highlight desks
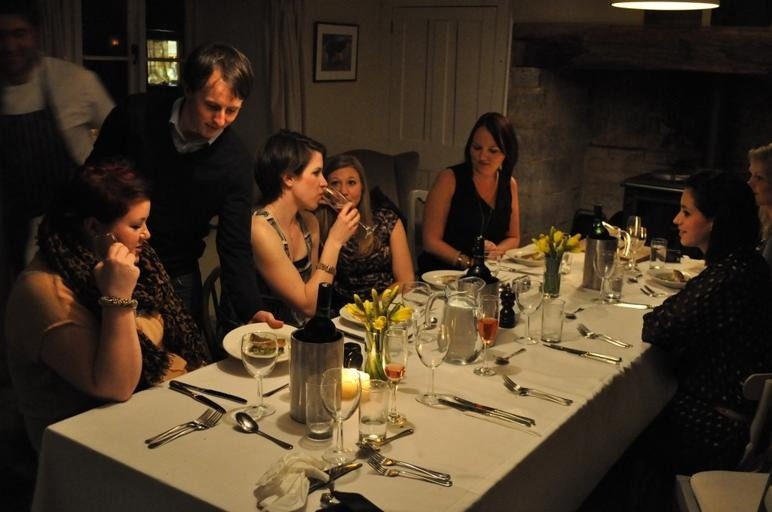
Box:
[623,170,695,247]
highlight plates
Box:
[222,321,301,364]
[421,270,466,290]
[645,267,688,289]
[505,248,544,267]
[339,303,366,327]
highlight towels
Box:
[253,450,330,511]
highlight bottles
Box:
[590,202,610,239]
[302,281,337,342]
[467,231,492,285]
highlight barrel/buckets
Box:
[583,236,618,289]
[288,329,343,424]
[459,276,500,321]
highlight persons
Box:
[619,171,772,512]
[82,43,284,329]
[311,153,416,319]
[746,142,772,268]
[417,112,521,283]
[212,127,361,361]
[2,163,209,453]
[0,0,118,298]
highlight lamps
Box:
[605,0,723,12]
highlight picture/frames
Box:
[312,21,362,82]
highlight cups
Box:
[599,262,625,304]
[559,233,572,274]
[649,237,668,269]
[540,298,566,344]
[358,379,393,447]
[304,372,337,444]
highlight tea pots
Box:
[424,276,487,366]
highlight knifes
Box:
[453,395,536,425]
[542,342,622,365]
[438,397,531,428]
[176,382,248,404]
[613,301,662,310]
[307,463,362,493]
[169,380,227,415]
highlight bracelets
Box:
[98,296,139,310]
[455,251,476,271]
[315,262,338,276]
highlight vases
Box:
[361,330,388,390]
[542,256,562,300]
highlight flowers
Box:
[530,225,583,294]
[344,285,415,381]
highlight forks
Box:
[368,458,453,487]
[501,373,574,404]
[502,381,570,406]
[148,408,223,450]
[143,408,213,445]
[577,322,633,349]
[364,444,450,481]
[640,285,672,299]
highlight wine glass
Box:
[241,331,279,418]
[514,278,543,346]
[320,186,378,238]
[319,368,362,467]
[381,329,409,426]
[415,323,450,406]
[627,215,642,228]
[402,281,433,345]
[591,248,616,305]
[90,233,120,264]
[473,295,500,377]
[623,227,648,275]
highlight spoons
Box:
[496,348,527,365]
[564,307,584,320]
[628,273,644,283]
[354,427,414,450]
[234,412,294,452]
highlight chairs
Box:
[405,186,430,271]
[675,365,772,511]
[196,261,225,345]
